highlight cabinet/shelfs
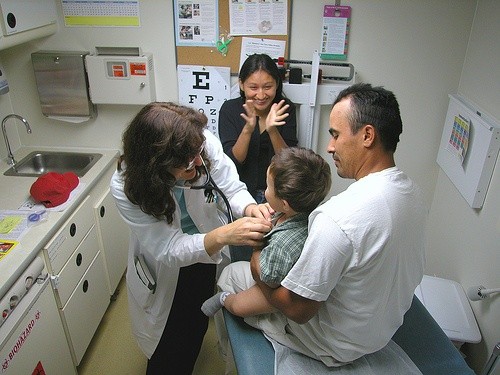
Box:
[41,160,130,369]
[0,0,59,51]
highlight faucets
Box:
[1,113,33,173]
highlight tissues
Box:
[31,49,99,124]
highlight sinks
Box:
[3,150,104,179]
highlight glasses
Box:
[170,138,206,171]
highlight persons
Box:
[109,101,276,375]
[218,52,300,262]
[217,83,425,369]
[201,146,331,318]
